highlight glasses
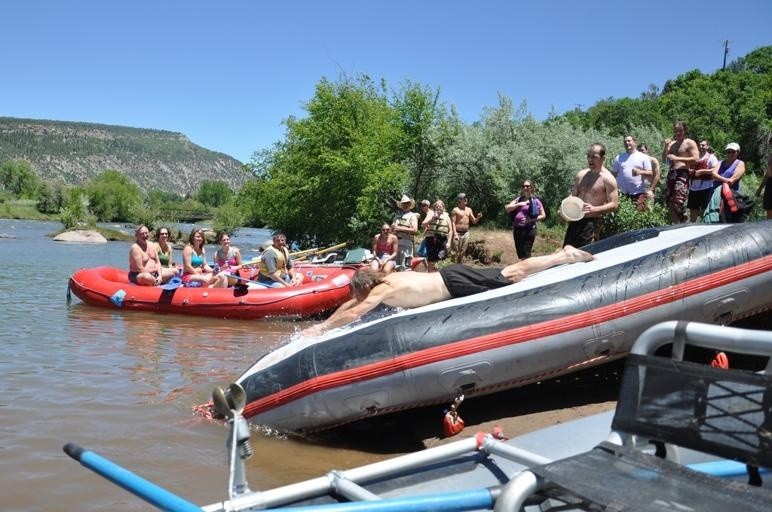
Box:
[522,185,531,189]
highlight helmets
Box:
[559,196,587,222]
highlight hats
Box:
[726,143,739,151]
[419,200,431,207]
[458,192,469,199]
[397,196,416,210]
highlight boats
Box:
[68,264,358,319]
[213,220,770,439]
[167,370,772,509]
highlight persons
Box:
[609,115,771,225]
[370,191,484,274]
[298,242,595,341]
[128,224,305,290]
[563,143,619,248]
[505,179,547,260]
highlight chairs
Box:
[491,317,771,511]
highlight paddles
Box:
[156,281,183,291]
